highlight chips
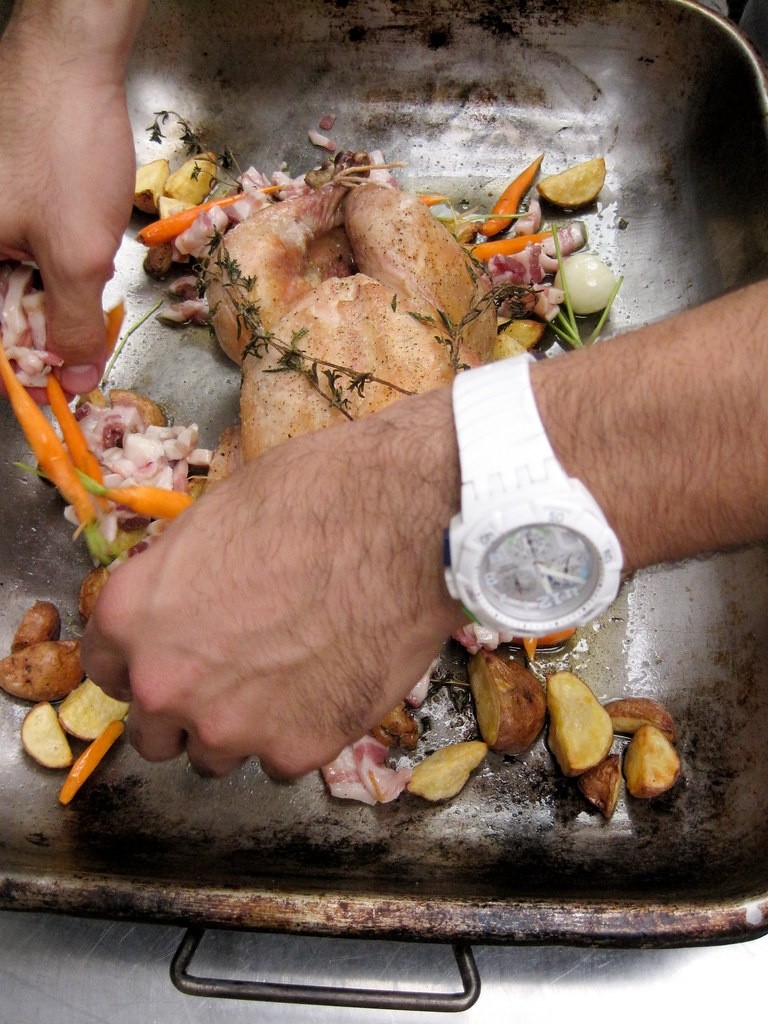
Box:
[132,146,219,215]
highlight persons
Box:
[0,2,768,788]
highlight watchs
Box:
[443,350,628,650]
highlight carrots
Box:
[0,296,207,566]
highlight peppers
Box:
[466,150,557,263]
[137,183,288,243]
[57,721,128,805]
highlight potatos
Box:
[406,647,679,820]
[0,598,133,770]
